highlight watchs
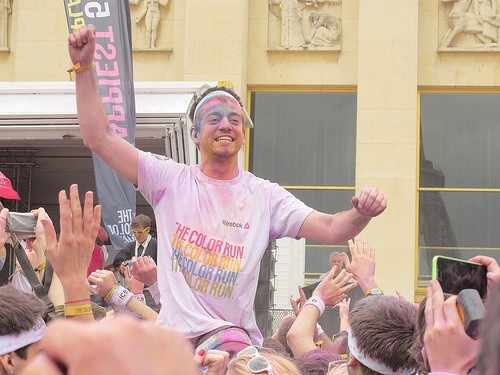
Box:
[363,288,383,297]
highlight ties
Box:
[138,246,144,257]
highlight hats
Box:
[97,226,108,243]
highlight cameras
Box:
[5,212,38,234]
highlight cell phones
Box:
[432,255,488,302]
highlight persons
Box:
[0,173,500,375]
[67,24,387,346]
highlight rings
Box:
[0,216,6,221]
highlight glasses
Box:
[130,227,148,235]
[236,346,274,375]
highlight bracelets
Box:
[67,61,95,81]
[104,285,117,303]
[64,305,92,316]
[65,297,91,304]
[35,260,46,273]
